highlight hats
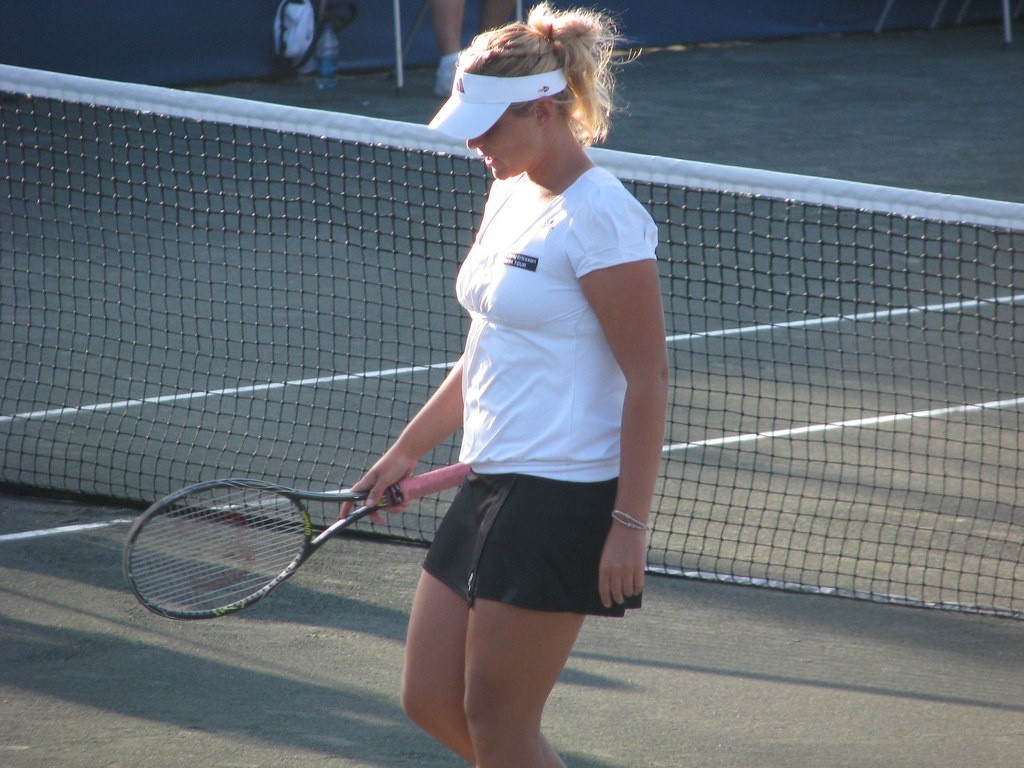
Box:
[426,64,570,142]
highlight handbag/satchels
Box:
[273,0,358,76]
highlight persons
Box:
[341,0,670,768]
[430,0,515,97]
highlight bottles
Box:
[315,21,340,89]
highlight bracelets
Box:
[611,510,651,530]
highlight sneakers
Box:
[433,67,456,97]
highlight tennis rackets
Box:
[125,458,478,623]
